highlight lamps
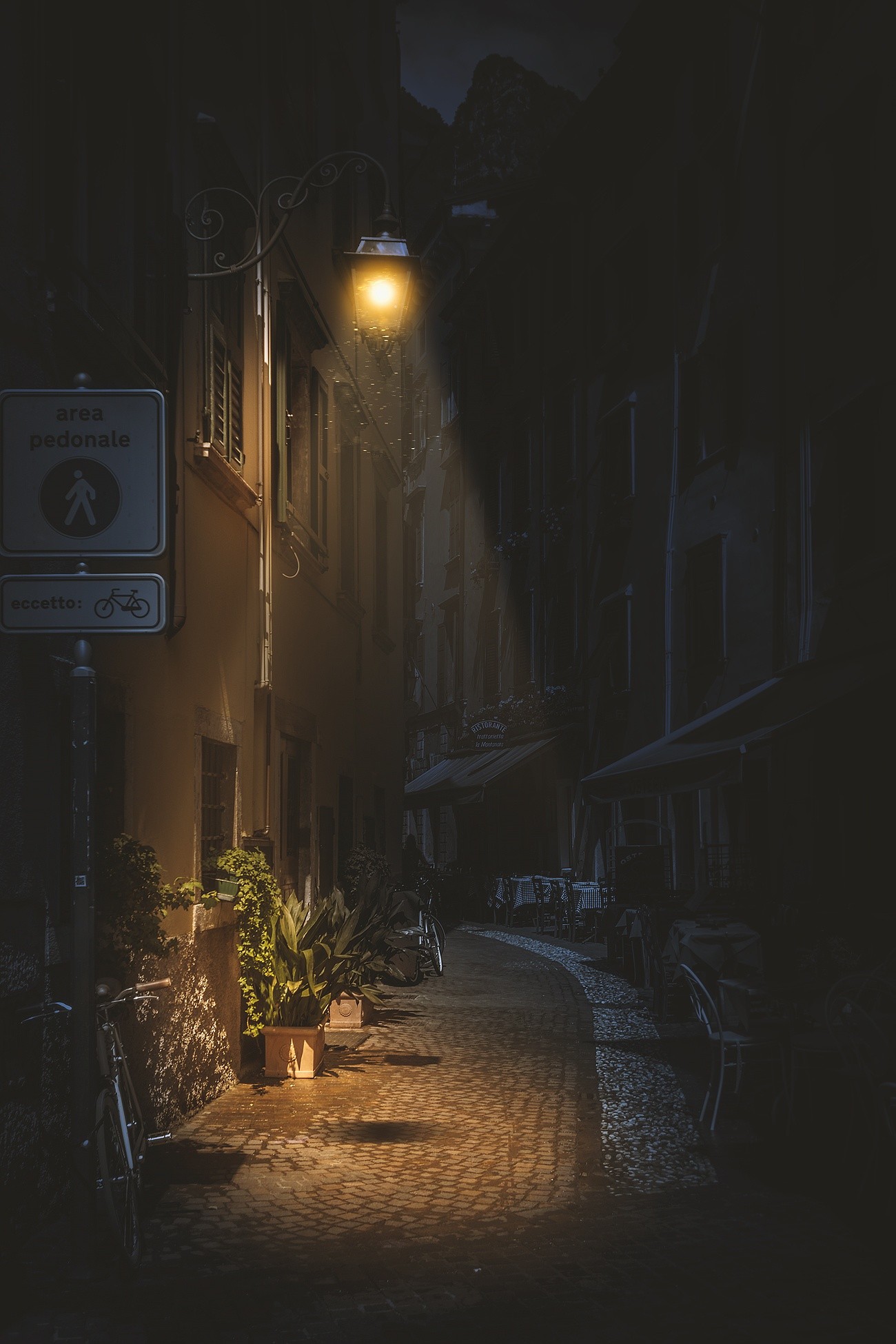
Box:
[153,146,424,367]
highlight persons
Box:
[401,834,429,888]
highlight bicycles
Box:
[15,978,173,1271]
[406,880,446,984]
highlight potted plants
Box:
[258,883,379,1075]
[312,883,409,1028]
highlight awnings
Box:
[405,734,567,804]
[580,676,819,805]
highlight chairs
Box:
[503,876,615,944]
[620,903,896,1133]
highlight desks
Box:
[488,875,616,941]
[715,978,781,1011]
[671,919,762,979]
